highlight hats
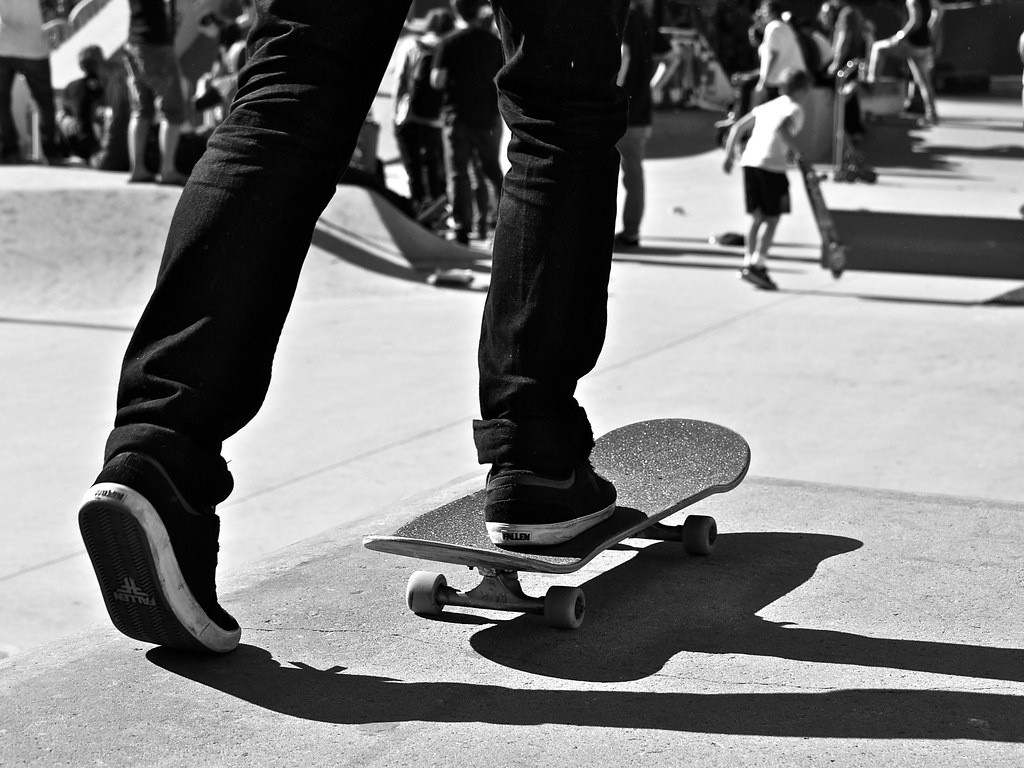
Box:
[407,7,455,36]
[78,46,101,65]
[199,13,226,28]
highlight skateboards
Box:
[362,418,751,629]
[796,153,847,278]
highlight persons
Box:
[77,0,625,650]
[0,0,943,251]
[722,67,812,290]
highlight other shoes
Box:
[740,264,775,290]
[126,171,156,182]
[446,231,494,247]
[916,113,937,124]
[156,171,186,185]
[613,231,639,252]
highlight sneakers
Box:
[482,461,619,550]
[76,452,242,659]
